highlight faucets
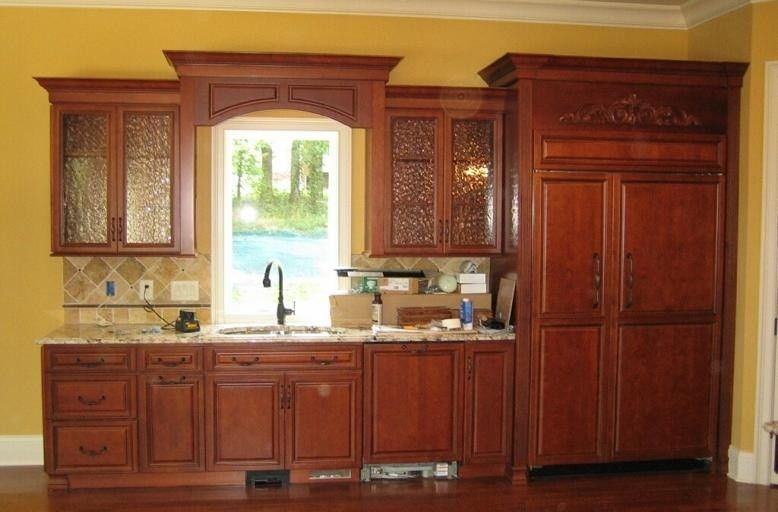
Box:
[263,261,296,325]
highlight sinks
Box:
[219,326,343,337]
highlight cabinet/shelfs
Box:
[478,52,751,487]
[140,344,205,472]
[463,339,516,464]
[204,342,363,471]
[32,78,181,257]
[362,340,466,466]
[383,84,502,256]
[39,344,139,474]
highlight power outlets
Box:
[139,279,153,300]
